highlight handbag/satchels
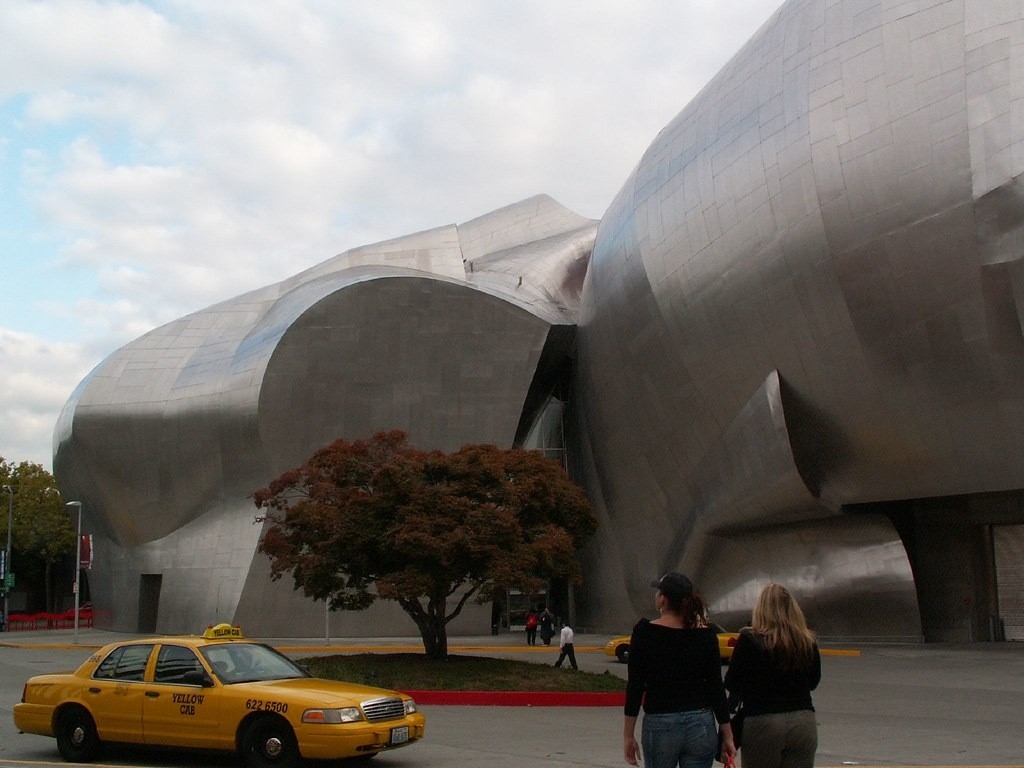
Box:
[526,614,538,628]
[715,707,744,763]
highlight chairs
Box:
[213,660,229,672]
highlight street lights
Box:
[67,501,82,631]
[2,484,13,631]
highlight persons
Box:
[524,613,538,646]
[554,620,578,670]
[724,582,822,768]
[623,572,737,768]
[540,606,555,646]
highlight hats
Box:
[650,573,693,598]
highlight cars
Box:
[604,620,742,664]
[13,624,425,768]
